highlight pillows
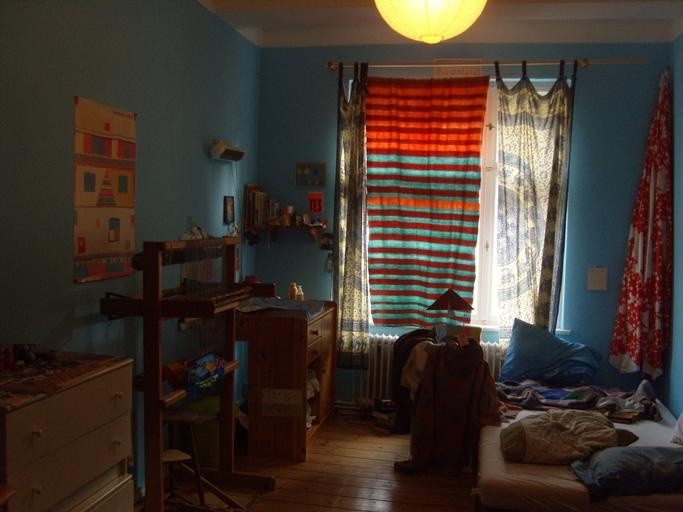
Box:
[498,319,614,385]
[501,410,683,500]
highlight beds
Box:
[475,381,683,510]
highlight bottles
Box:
[287,280,304,301]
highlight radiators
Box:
[353,335,508,414]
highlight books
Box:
[333,397,398,424]
[241,181,282,228]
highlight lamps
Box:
[374,1,488,45]
